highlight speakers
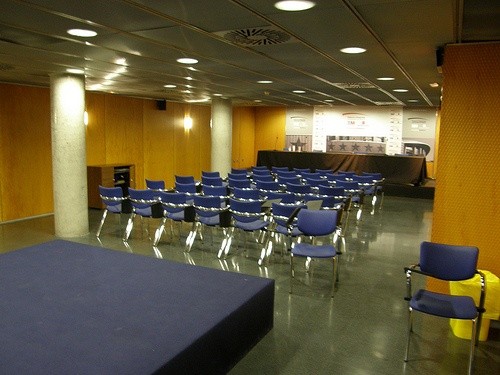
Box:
[158,100,166,110]
[435,46,444,67]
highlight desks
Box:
[257,150,427,187]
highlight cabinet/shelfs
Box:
[87,164,135,209]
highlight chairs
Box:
[403,241,485,375]
[96,167,385,297]
